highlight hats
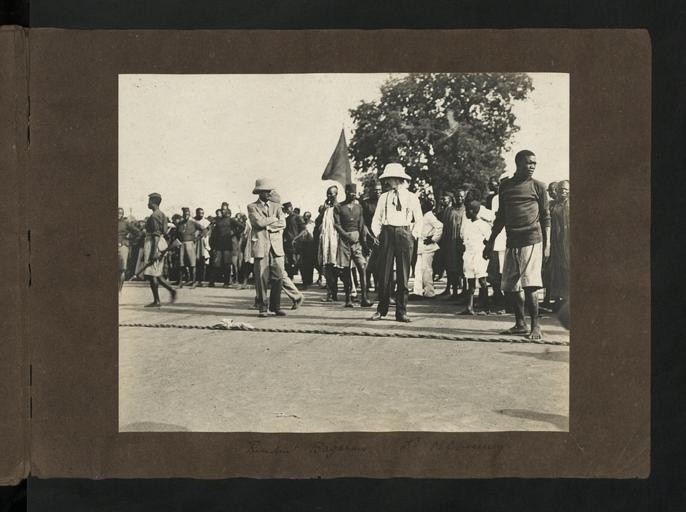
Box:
[252,179,279,194]
[378,163,411,180]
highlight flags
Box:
[322,130,354,192]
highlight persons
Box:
[484,150,552,340]
[367,162,423,323]
[119,179,570,325]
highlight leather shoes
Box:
[274,309,286,316]
[372,313,388,320]
[259,311,269,317]
[396,314,410,321]
